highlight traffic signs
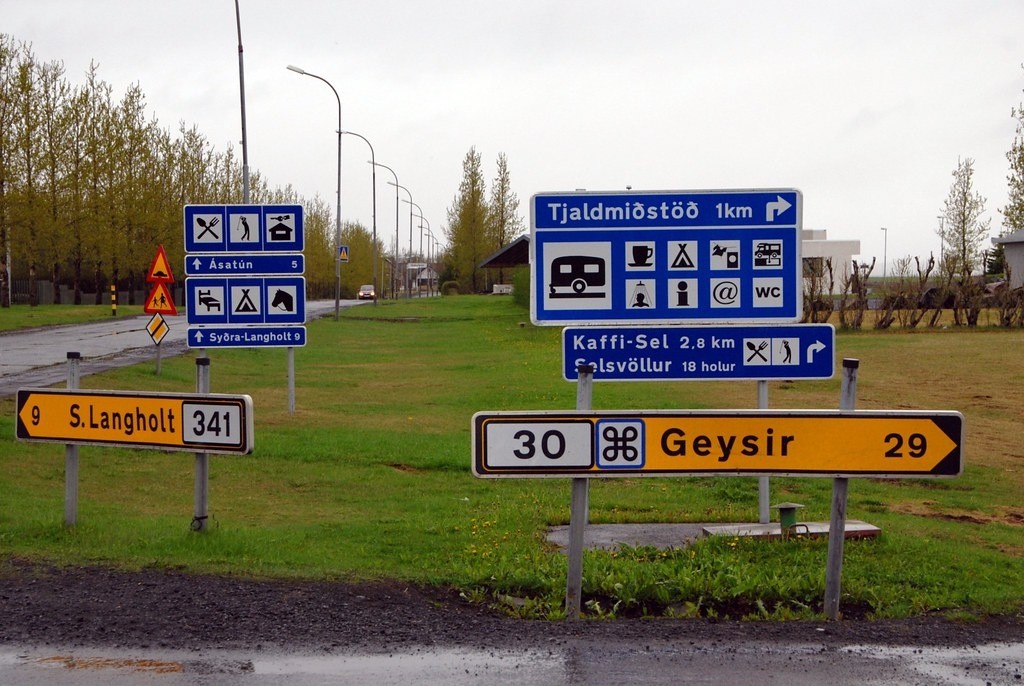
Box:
[469,411,966,479]
[562,325,836,382]
[187,326,306,348]
[14,388,254,455]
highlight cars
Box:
[357,285,375,300]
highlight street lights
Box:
[368,160,398,300]
[336,130,377,306]
[424,234,439,297]
[402,200,423,297]
[417,225,434,298]
[286,65,342,320]
[387,182,412,299]
[412,214,430,298]
[435,243,445,251]
